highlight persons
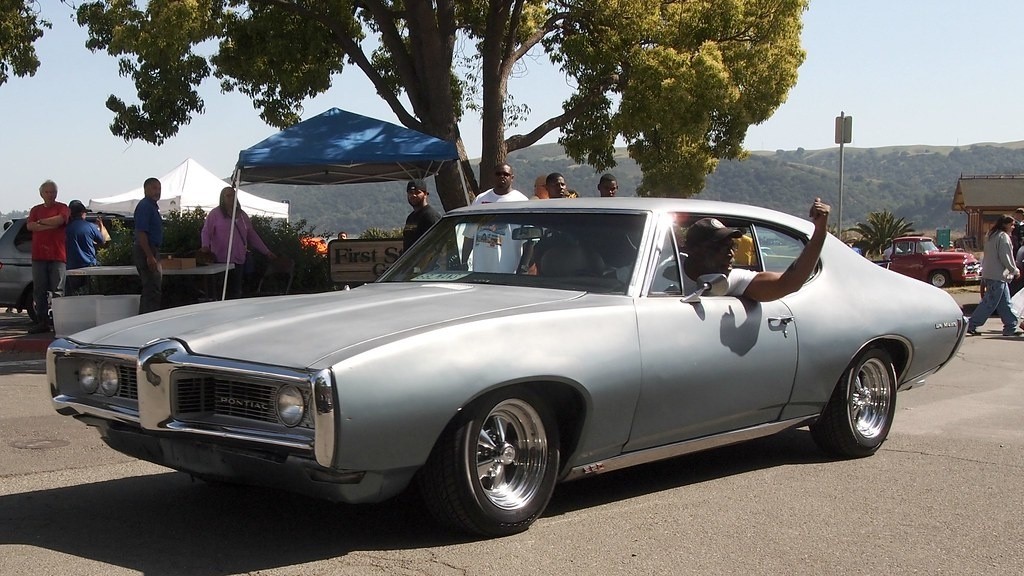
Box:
[530,173,618,276]
[882,238,902,260]
[462,163,540,276]
[402,179,442,276]
[27,180,70,333]
[64,200,105,295]
[338,232,348,240]
[201,187,278,299]
[601,198,831,302]
[965,207,1024,336]
[133,178,165,314]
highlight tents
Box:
[90,158,289,229]
[221,108,471,301]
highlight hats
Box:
[71,203,92,212]
[407,180,429,196]
[686,217,743,250]
[1016,208,1024,216]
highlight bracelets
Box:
[519,264,529,271]
[38,220,42,224]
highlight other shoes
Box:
[28,315,50,333]
[967,329,981,335]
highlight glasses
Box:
[496,171,511,177]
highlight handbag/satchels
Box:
[245,248,255,268]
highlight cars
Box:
[41,196,974,552]
[0,214,190,323]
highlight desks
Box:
[65,264,235,302]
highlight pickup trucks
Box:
[861,233,981,286]
